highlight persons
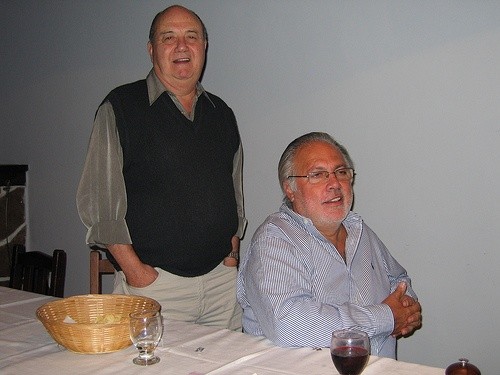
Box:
[237,132,423,359]
[74,4,248,335]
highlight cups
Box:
[330,330,370,375]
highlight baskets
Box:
[36,294,161,355]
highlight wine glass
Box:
[128,309,162,366]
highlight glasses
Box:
[287,167,356,186]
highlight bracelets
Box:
[225,253,239,260]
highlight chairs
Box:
[89,251,116,294]
[10,244,67,298]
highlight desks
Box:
[0,285,447,375]
